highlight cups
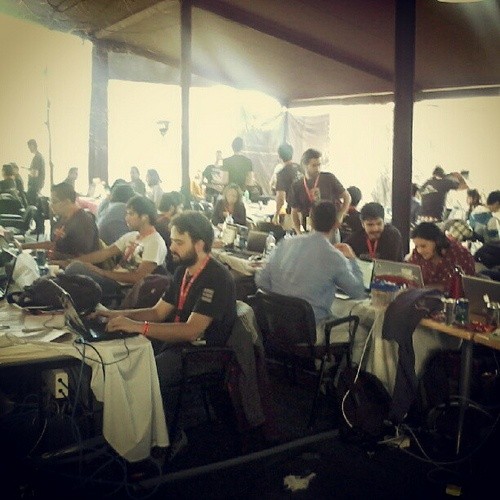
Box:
[487,302,500,330]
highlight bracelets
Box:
[143,321,148,336]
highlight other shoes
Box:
[169,429,188,461]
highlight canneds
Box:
[456,298,469,323]
[446,302,455,326]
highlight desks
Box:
[0,244,500,500]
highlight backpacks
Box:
[7,273,102,313]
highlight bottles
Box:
[265,231,276,259]
[284,231,292,240]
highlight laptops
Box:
[375,260,424,289]
[218,225,238,247]
[334,258,375,299]
[50,278,140,343]
[0,248,17,300]
[229,231,269,260]
[461,275,500,328]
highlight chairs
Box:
[98,220,500,443]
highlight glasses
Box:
[47,200,62,207]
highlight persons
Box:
[288,148,351,245]
[99,166,186,275]
[49,193,168,308]
[65,167,81,197]
[22,182,101,270]
[408,167,467,228]
[467,190,500,283]
[22,139,45,234]
[343,202,403,262]
[340,186,365,242]
[87,210,236,480]
[405,222,475,283]
[256,199,369,394]
[269,143,304,231]
[200,137,253,203]
[212,184,246,225]
[0,163,24,193]
